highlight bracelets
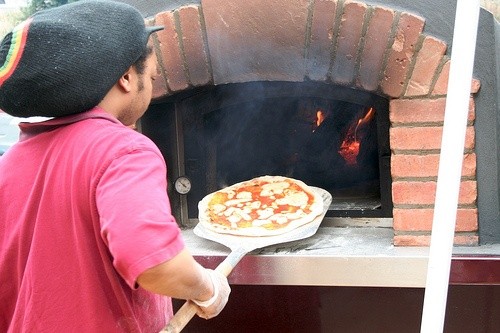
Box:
[186,271,218,308]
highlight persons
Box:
[0,1,231,333]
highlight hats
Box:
[0,0,165,117]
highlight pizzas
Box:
[197,174,325,237]
